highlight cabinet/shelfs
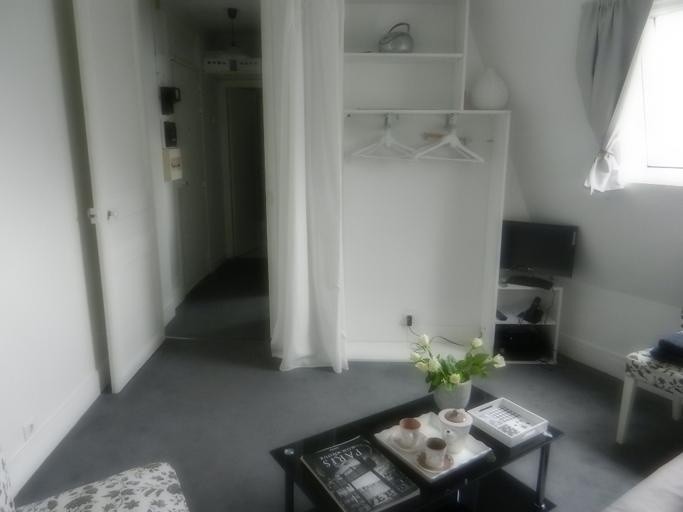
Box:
[493,278,564,365]
[341,0,469,111]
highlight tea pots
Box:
[436,407,474,455]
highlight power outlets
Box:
[404,312,415,327]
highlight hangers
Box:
[415,114,485,162]
[350,114,418,161]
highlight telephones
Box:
[524,297,544,323]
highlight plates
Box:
[387,429,428,454]
[414,451,455,474]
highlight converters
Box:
[406,316,412,326]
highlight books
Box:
[300,433,422,511]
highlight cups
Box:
[424,437,448,468]
[399,417,422,448]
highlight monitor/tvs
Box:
[500,220,578,289]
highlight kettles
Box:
[378,21,414,55]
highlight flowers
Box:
[410,335,505,393]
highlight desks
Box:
[269,385,565,512]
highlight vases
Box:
[433,380,472,410]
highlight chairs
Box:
[0,453,190,512]
[615,308,682,444]
